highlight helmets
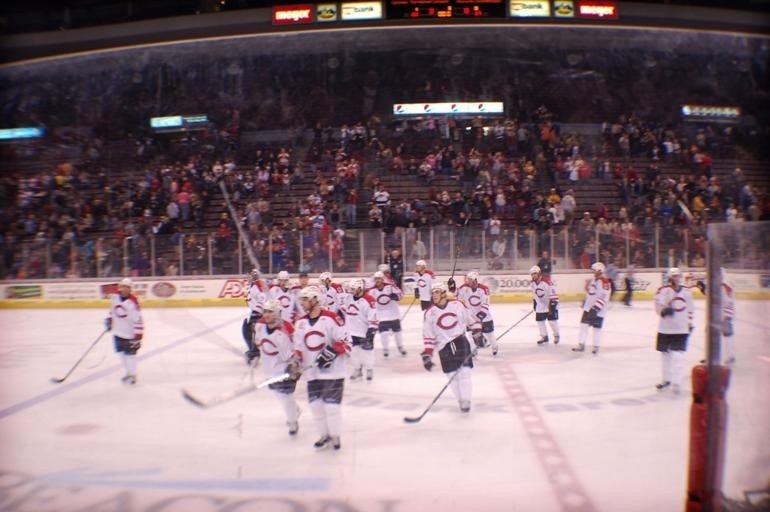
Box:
[374,271,384,278]
[351,279,364,291]
[416,260,426,266]
[668,268,682,278]
[300,285,323,302]
[120,277,133,287]
[320,272,332,282]
[431,283,447,293]
[530,266,541,275]
[262,300,283,312]
[276,271,290,282]
[468,272,478,280]
[591,262,606,273]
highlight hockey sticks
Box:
[51,328,111,384]
[183,360,321,409]
[404,344,483,422]
[482,309,535,348]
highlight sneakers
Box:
[384,349,387,356]
[122,374,134,383]
[571,344,599,353]
[366,370,372,380]
[459,400,470,412]
[399,347,406,354]
[554,336,559,343]
[537,336,548,344]
[315,436,340,449]
[289,422,299,434]
[350,369,360,379]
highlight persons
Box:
[529,266,561,345]
[653,268,695,393]
[572,263,611,352]
[695,266,736,365]
[242,265,407,450]
[105,278,144,385]
[0,97,770,285]
[413,260,500,411]
[606,262,634,306]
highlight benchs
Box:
[0,100,768,276]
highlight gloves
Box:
[476,311,486,321]
[389,293,398,300]
[105,318,112,331]
[587,306,600,318]
[472,331,487,347]
[548,300,557,315]
[317,345,338,368]
[284,360,301,383]
[661,308,675,316]
[366,328,377,342]
[129,339,140,351]
[421,352,433,371]
